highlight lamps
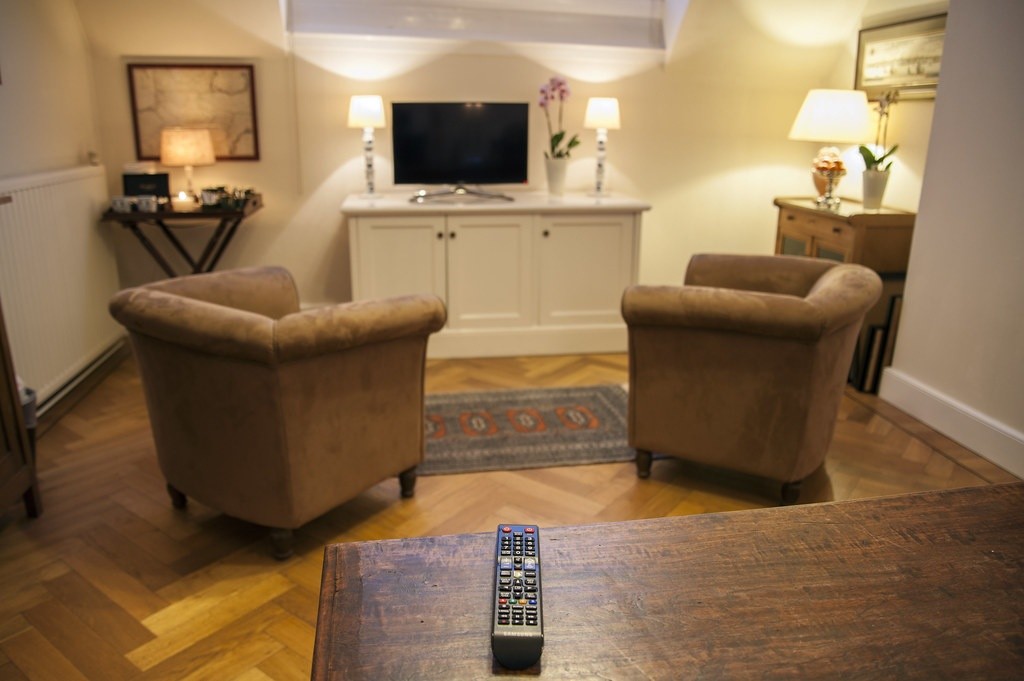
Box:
[582,97,626,198]
[780,86,881,212]
[349,93,387,204]
[160,127,218,206]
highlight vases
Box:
[862,171,891,208]
[545,160,567,195]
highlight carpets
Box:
[399,381,675,485]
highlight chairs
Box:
[111,267,450,562]
[622,253,885,503]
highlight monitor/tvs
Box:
[392,101,529,205]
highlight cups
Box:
[113,195,159,215]
[202,186,226,204]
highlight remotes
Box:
[490,523,546,658]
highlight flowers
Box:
[534,74,579,159]
[859,90,897,170]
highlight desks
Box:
[107,194,265,278]
[309,459,1023,681]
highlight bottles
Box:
[220,193,230,210]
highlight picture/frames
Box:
[852,12,948,100]
[127,62,259,161]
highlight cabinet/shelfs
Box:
[344,191,652,359]
[772,198,915,393]
